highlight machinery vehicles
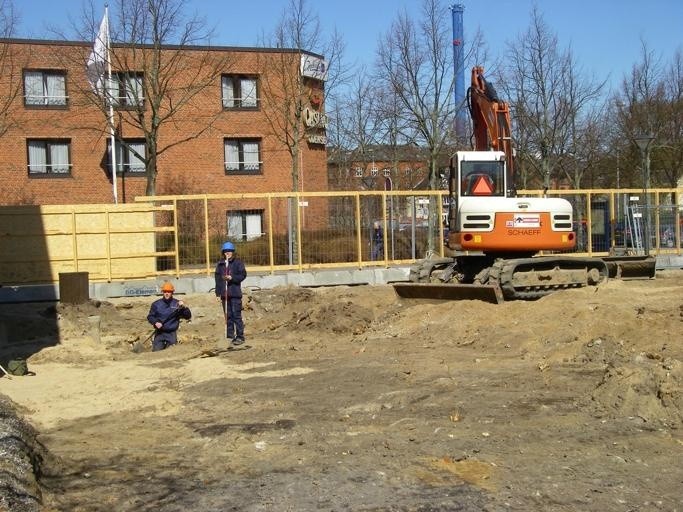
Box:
[391,66,656,305]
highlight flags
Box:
[85,13,111,95]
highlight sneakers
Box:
[233,338,244,345]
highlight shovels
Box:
[132,302,184,354]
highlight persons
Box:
[215,242,247,344]
[148,282,191,352]
[368,222,384,261]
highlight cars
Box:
[373,196,449,231]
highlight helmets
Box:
[222,242,235,251]
[161,282,174,292]
